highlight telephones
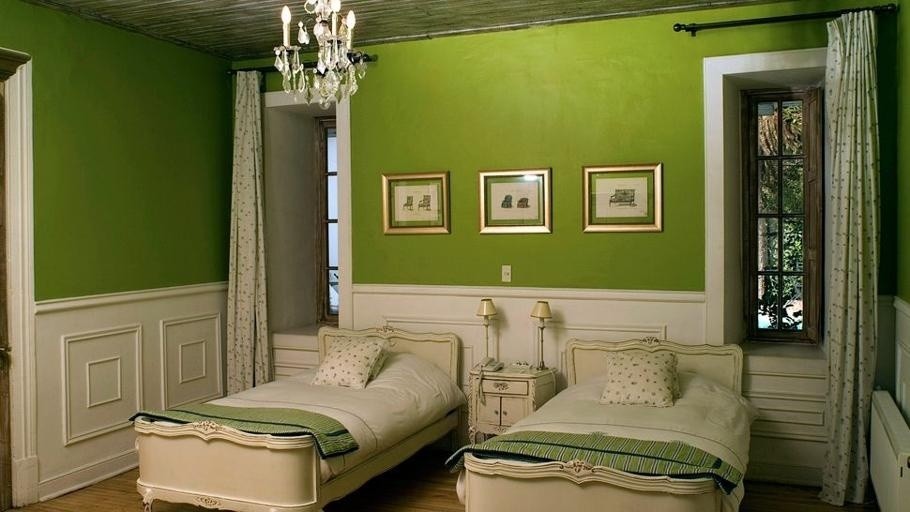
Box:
[476,357,504,372]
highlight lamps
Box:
[474,297,499,360]
[269,1,372,112]
[528,300,555,373]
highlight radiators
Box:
[862,383,910,511]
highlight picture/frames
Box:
[476,167,554,236]
[579,159,666,237]
[375,170,457,237]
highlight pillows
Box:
[670,354,683,402]
[598,347,675,409]
[366,347,391,381]
[308,336,382,391]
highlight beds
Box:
[437,336,752,512]
[120,321,470,511]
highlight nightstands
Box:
[467,357,562,444]
